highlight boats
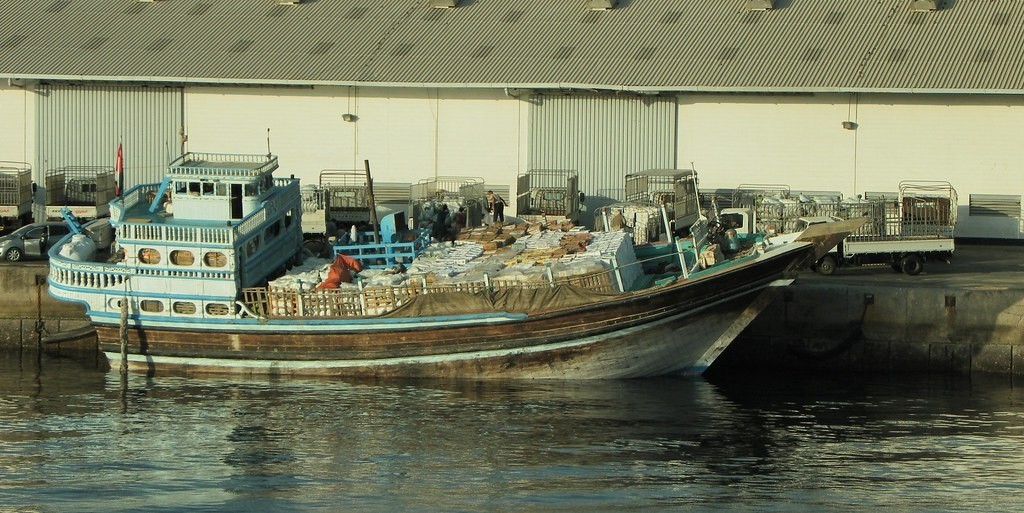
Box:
[43,127,866,376]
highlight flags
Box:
[114,143,124,196]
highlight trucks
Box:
[757,214,956,276]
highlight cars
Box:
[0,222,72,263]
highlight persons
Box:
[431,203,465,247]
[487,189,508,226]
[326,218,369,255]
